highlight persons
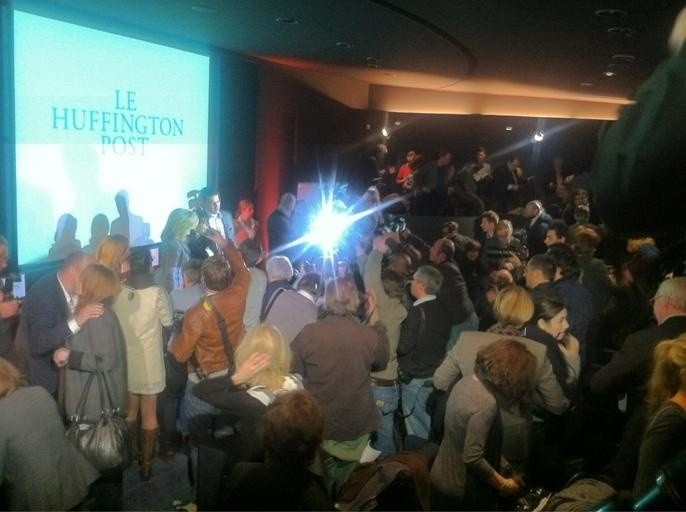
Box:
[2,138,685,512]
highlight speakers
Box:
[354,110,378,144]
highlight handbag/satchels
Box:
[66,414,127,469]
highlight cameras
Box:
[186,229,218,258]
[486,239,530,260]
[1,273,25,308]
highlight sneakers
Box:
[172,500,196,512]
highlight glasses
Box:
[649,296,664,305]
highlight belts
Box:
[370,377,399,387]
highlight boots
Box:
[124,420,142,464]
[140,427,160,480]
